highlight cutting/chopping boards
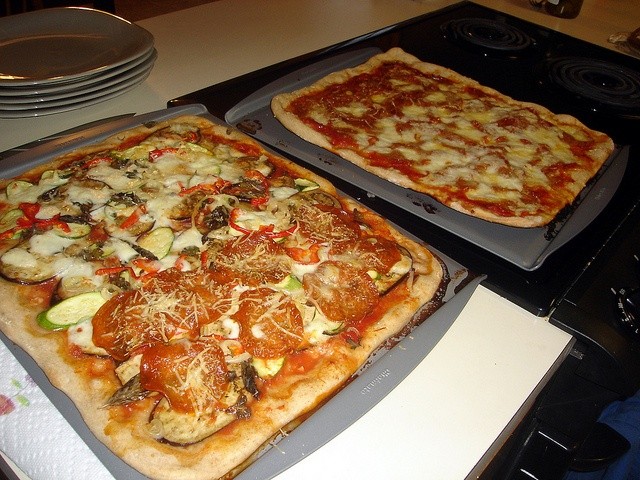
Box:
[225,47,629,272]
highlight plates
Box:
[0,7,158,120]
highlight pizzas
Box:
[272,46,616,233]
